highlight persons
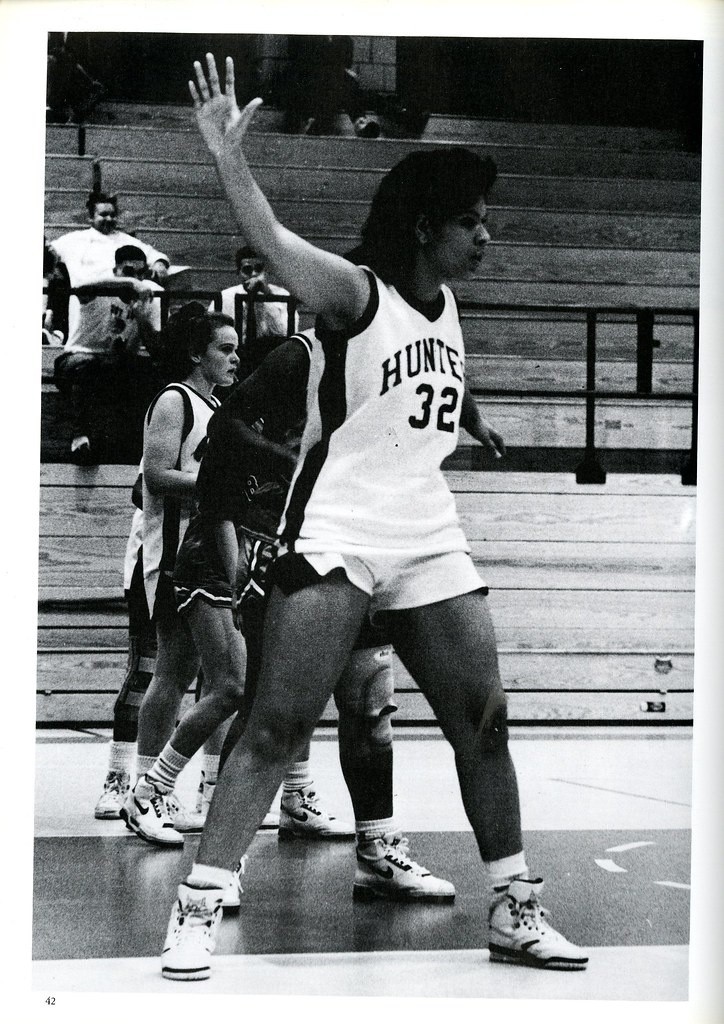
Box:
[161,51,590,980]
[42,194,457,911]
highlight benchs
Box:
[41,99,700,471]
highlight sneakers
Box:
[489,878,589,971]
[353,829,456,903]
[222,852,249,909]
[202,783,217,815]
[196,770,205,812]
[119,773,185,848]
[161,884,224,981]
[278,781,357,839]
[164,793,207,829]
[95,771,131,819]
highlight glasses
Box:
[240,263,266,275]
[118,264,147,277]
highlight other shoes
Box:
[71,435,92,453]
